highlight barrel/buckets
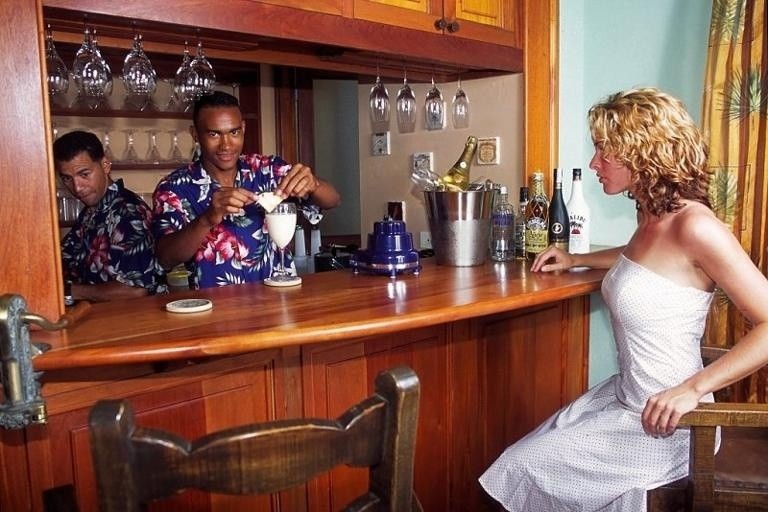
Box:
[418,170,501,267]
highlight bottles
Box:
[490,167,590,262]
[438,136,478,191]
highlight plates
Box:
[263,276,303,287]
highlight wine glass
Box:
[425,67,444,131]
[367,58,390,131]
[44,13,216,102]
[264,201,300,283]
[452,71,469,129]
[50,120,182,163]
[392,62,417,133]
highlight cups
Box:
[57,196,84,223]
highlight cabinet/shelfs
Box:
[353,0,524,53]
[42,31,251,289]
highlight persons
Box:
[54,131,167,290]
[153,91,343,290]
[478,87,768,512]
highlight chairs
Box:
[92,360,419,510]
[650,304,766,512]
